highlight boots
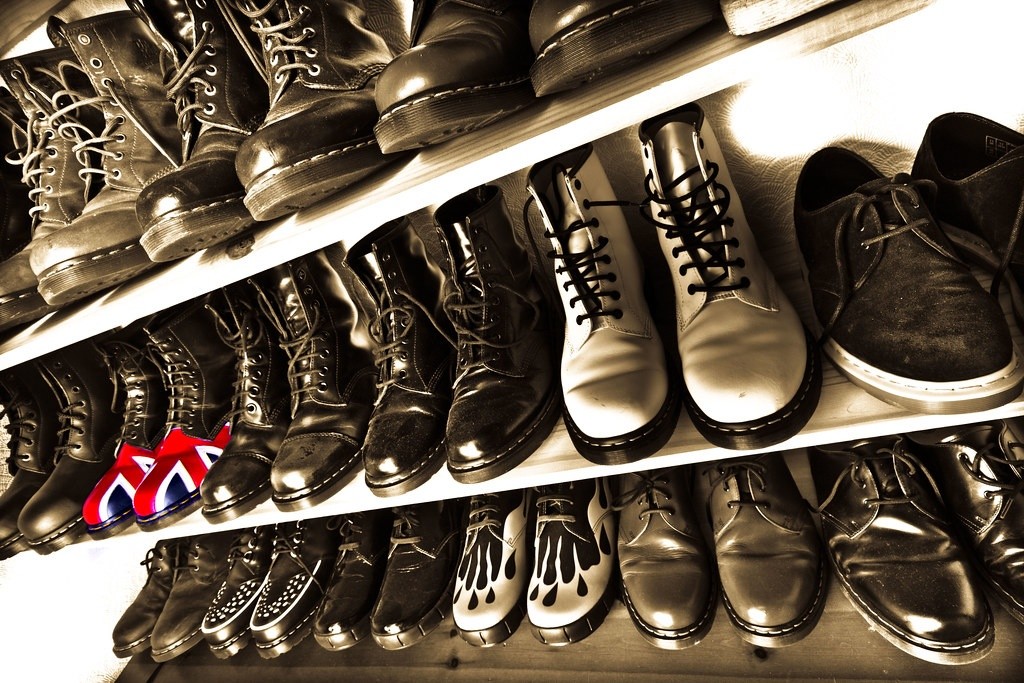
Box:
[0,0,1024,683]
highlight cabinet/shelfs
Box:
[0,1,1023,683]
